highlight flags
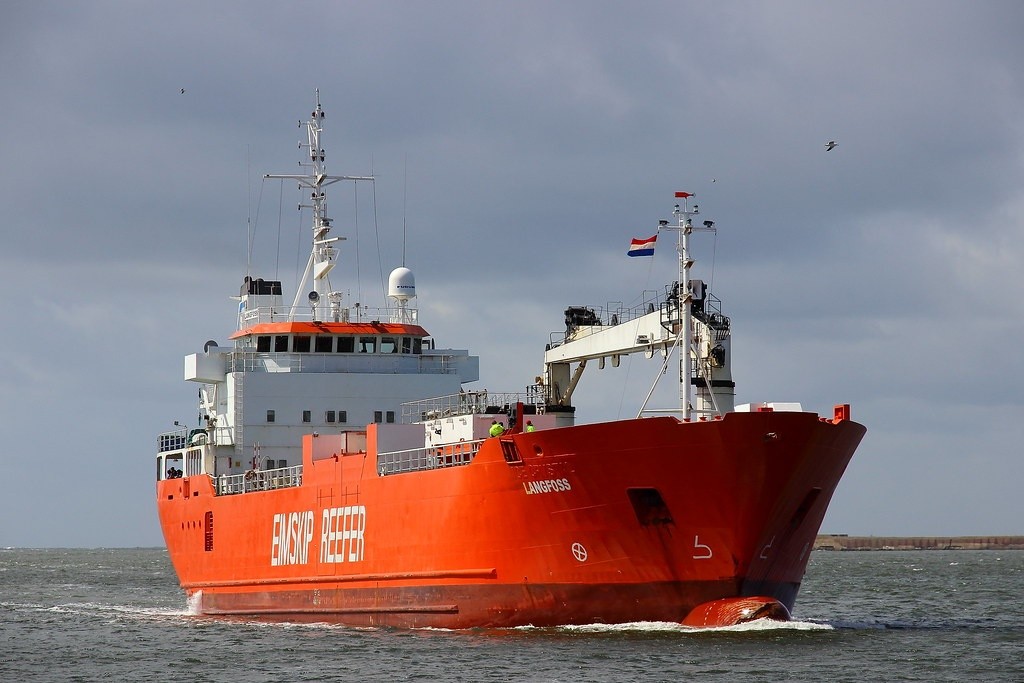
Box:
[627,235,658,258]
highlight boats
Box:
[155,85,869,639]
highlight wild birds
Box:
[825,141,839,152]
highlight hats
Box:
[499,422,503,426]
[525,420,531,425]
[492,420,497,424]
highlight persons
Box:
[488,420,505,438]
[167,466,183,478]
[525,420,535,432]
